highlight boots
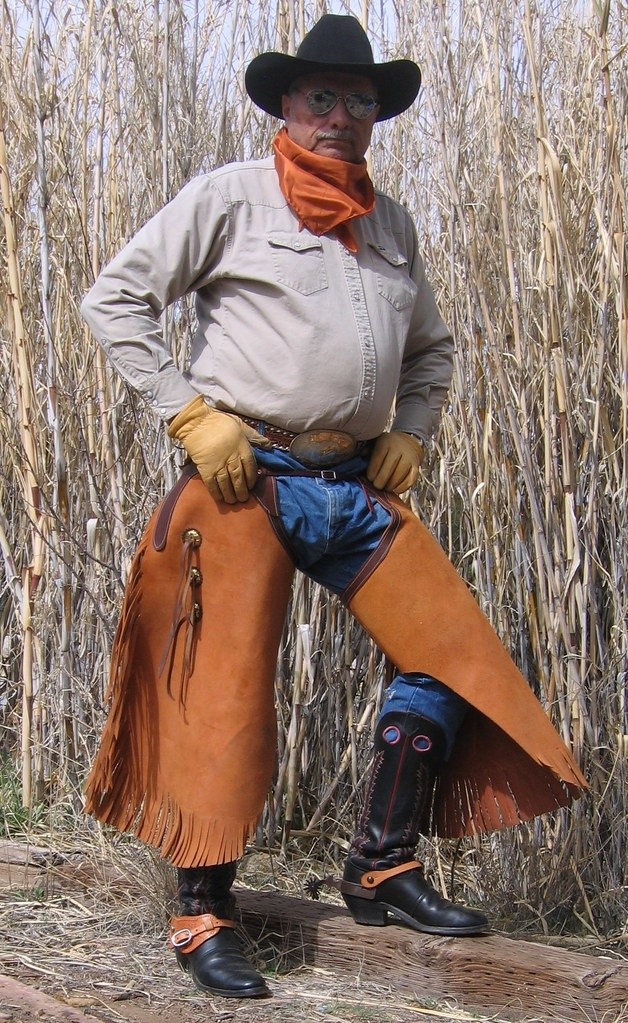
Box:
[340,712,491,937]
[169,858,274,999]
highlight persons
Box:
[77,15,587,995]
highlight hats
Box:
[245,14,422,122]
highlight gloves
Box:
[367,432,424,495]
[167,393,274,505]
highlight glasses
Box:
[290,86,381,121]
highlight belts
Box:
[222,409,375,472]
